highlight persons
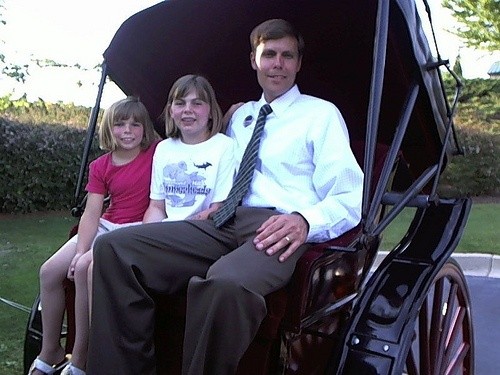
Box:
[86,75,236,331]
[82,19,364,374]
[27,97,245,375]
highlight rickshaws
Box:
[23,1,474,375]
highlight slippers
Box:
[59,365,86,375]
[26,353,71,374]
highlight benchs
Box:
[21,0,480,375]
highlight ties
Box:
[212,104,274,228]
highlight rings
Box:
[285,236,290,243]
